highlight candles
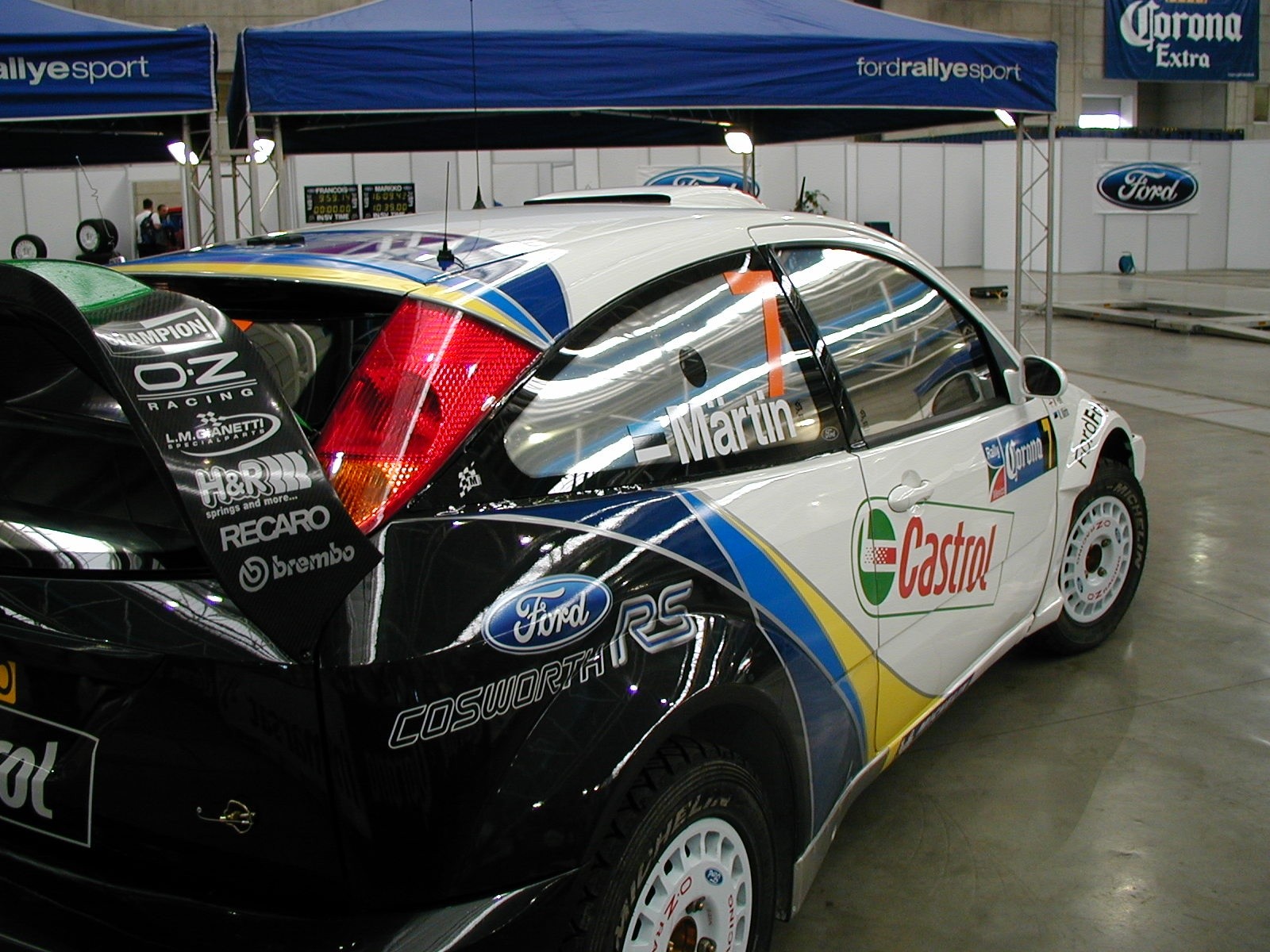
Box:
[11,234,47,259]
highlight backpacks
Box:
[140,212,156,248]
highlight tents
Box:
[0,0,229,254]
[237,1,1057,372]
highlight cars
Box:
[0,187,1151,952]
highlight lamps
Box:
[995,110,1018,127]
[244,121,276,165]
[166,118,200,165]
[723,109,755,155]
[76,218,120,265]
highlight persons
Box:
[135,198,163,258]
[154,205,180,254]
